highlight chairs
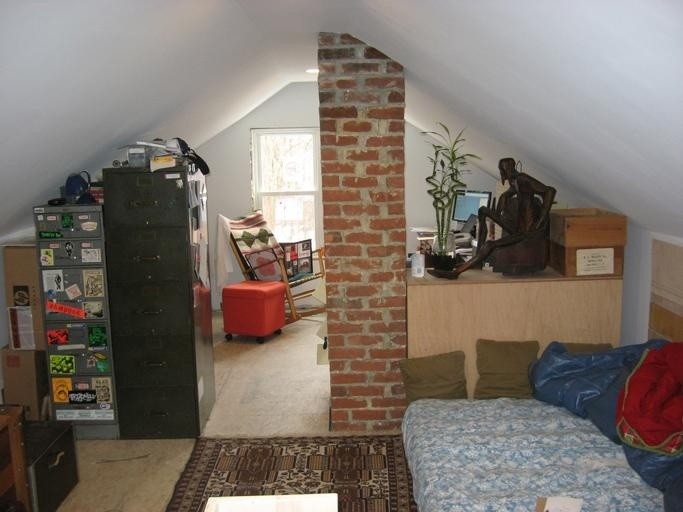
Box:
[217,208,326,325]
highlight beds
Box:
[398,302,682,511]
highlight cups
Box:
[411,255,425,278]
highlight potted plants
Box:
[418,121,484,268]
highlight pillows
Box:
[400,338,613,408]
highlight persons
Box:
[421,155,557,280]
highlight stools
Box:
[222,280,289,344]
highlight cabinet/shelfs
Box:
[103,168,219,441]
[31,204,118,428]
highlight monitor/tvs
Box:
[451,189,492,223]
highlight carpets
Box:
[164,432,418,512]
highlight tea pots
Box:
[60,170,91,204]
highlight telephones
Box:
[454,233,472,245]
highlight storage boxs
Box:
[548,206,628,279]
[0,239,48,421]
